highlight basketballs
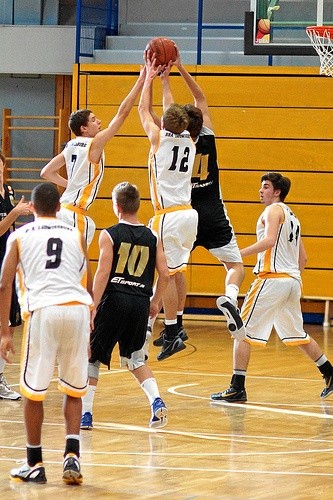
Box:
[145,37,178,69]
[257,19,271,34]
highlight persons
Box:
[210,173,333,406]
[0,149,32,401]
[80,181,169,429]
[139,50,247,361]
[0,182,94,486]
[40,65,147,248]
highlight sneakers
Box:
[153,326,188,347]
[8,460,47,484]
[211,383,246,403]
[0,372,22,401]
[80,412,93,429]
[62,453,83,485]
[146,327,153,341]
[216,295,246,342]
[149,398,168,428]
[157,334,186,361]
[320,373,333,398]
